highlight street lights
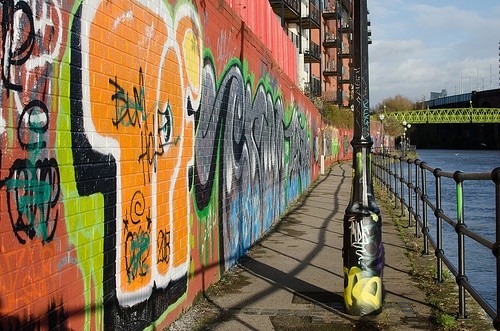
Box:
[402,121,411,157]
[379,115,385,160]
